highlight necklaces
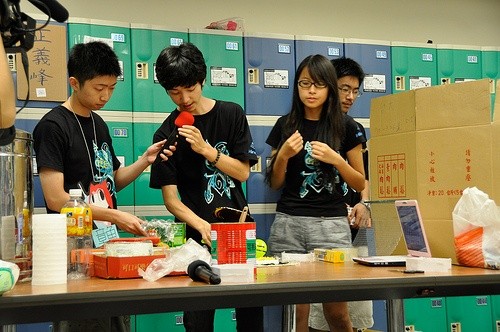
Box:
[67,97,102,184]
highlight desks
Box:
[0,246,500,332]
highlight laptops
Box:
[352,200,431,266]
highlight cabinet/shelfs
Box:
[10,13,500,332]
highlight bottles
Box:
[22,190,33,259]
[59,189,94,280]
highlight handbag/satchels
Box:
[452,187,500,269]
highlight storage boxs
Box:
[92,251,190,279]
[406,256,452,271]
[313,245,346,264]
[366,75,500,267]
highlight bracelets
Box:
[361,200,370,209]
[208,148,220,165]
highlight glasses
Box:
[337,87,362,97]
[296,80,329,88]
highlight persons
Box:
[265,54,366,332]
[300,56,374,332]
[33,41,177,332]
[148,43,264,332]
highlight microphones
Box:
[151,111,195,169]
[27,0,70,23]
[187,260,221,284]
